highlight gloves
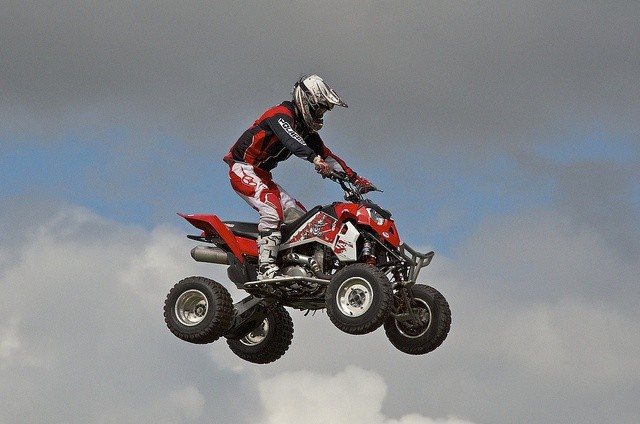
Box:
[355,177,376,193]
[314,155,331,179]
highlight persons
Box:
[223,74,377,281]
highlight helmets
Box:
[291,74,348,130]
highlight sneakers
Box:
[257,264,290,280]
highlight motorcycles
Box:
[165,165,451,364]
